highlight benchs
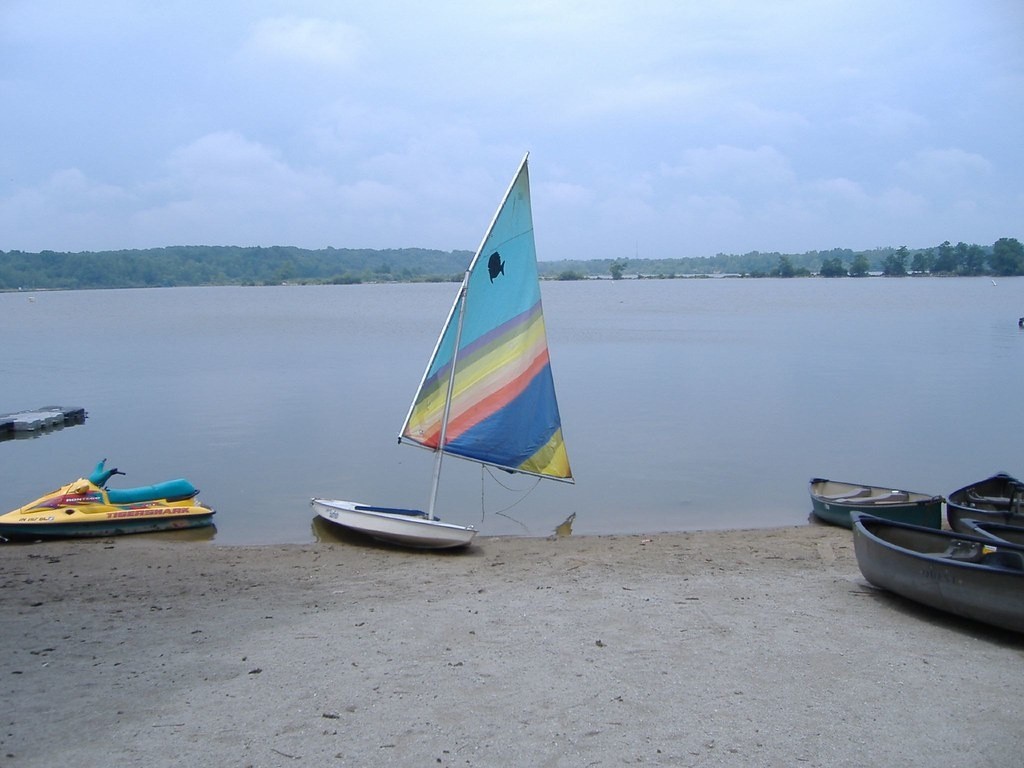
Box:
[968,489,1024,511]
[817,486,871,500]
[103,478,200,504]
[922,539,983,563]
[354,506,440,522]
[830,491,910,505]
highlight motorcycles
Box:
[0,457,219,543]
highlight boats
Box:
[846,507,1023,636]
[957,516,1023,544]
[947,468,1024,527]
[807,474,944,532]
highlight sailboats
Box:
[307,147,578,553]
